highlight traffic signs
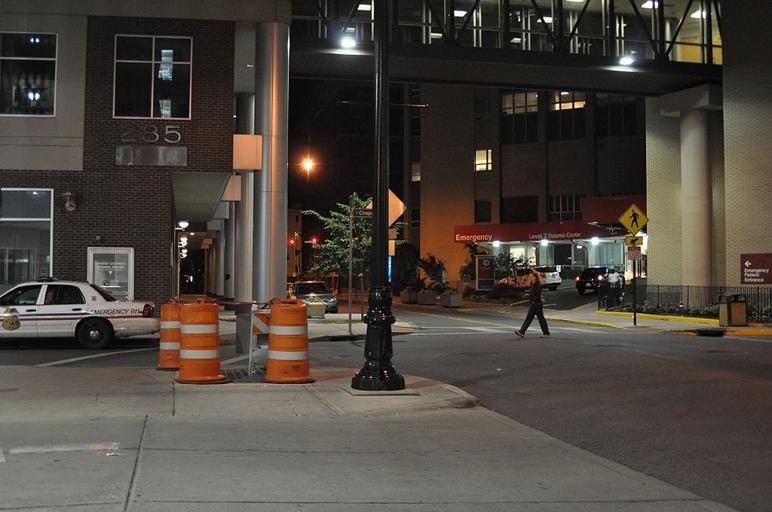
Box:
[741,252,772,286]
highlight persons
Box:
[514,273,551,338]
[608,269,620,305]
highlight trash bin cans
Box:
[325,275,339,296]
[235,302,258,355]
[719,293,748,327]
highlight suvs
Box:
[499,265,562,292]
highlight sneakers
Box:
[514,331,524,337]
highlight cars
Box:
[575,265,627,295]
[288,281,338,316]
[1,275,160,350]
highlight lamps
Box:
[175,220,190,260]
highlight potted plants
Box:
[300,294,329,319]
